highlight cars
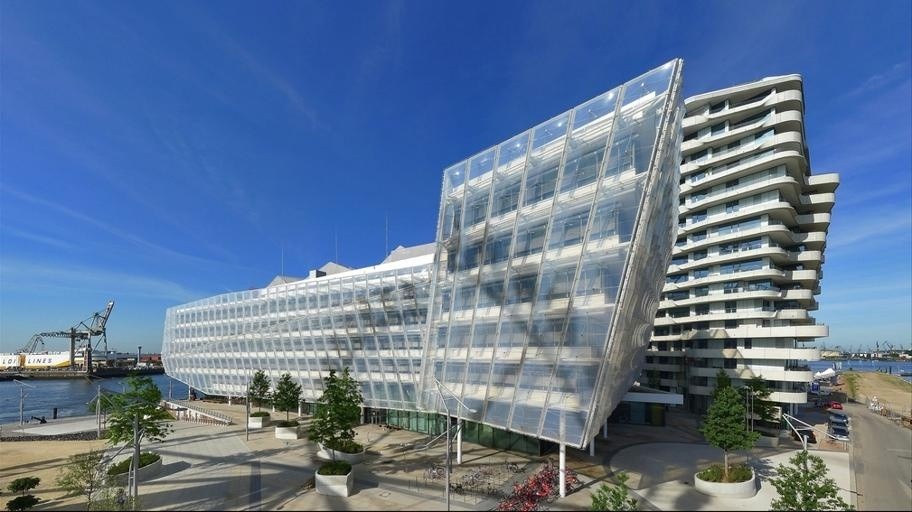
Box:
[825,399,849,441]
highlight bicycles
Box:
[420,454,582,511]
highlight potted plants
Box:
[90,367,176,488]
[246,366,365,498]
[694,366,758,498]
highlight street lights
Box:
[780,411,852,459]
[96,386,188,511]
[431,375,478,512]
